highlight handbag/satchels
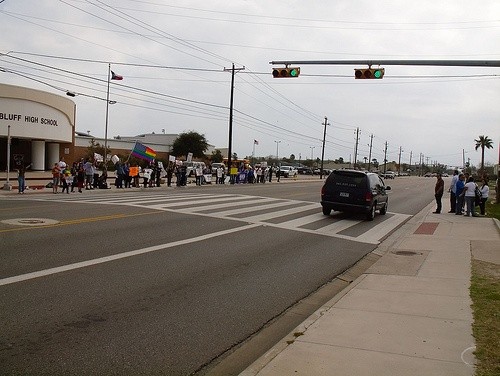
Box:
[475,184,482,197]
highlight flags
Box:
[254,139,259,145]
[111,70,123,80]
[131,141,156,163]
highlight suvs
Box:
[320,168,392,221]
[371,169,410,179]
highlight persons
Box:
[447,170,459,214]
[57,156,66,188]
[432,172,444,214]
[71,157,284,195]
[52,163,59,194]
[495,178,498,202]
[13,162,33,194]
[459,177,477,217]
[62,164,71,194]
[478,179,489,216]
[456,174,465,215]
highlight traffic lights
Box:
[272,67,300,78]
[354,68,385,79]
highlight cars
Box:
[178,158,369,177]
[423,172,449,177]
